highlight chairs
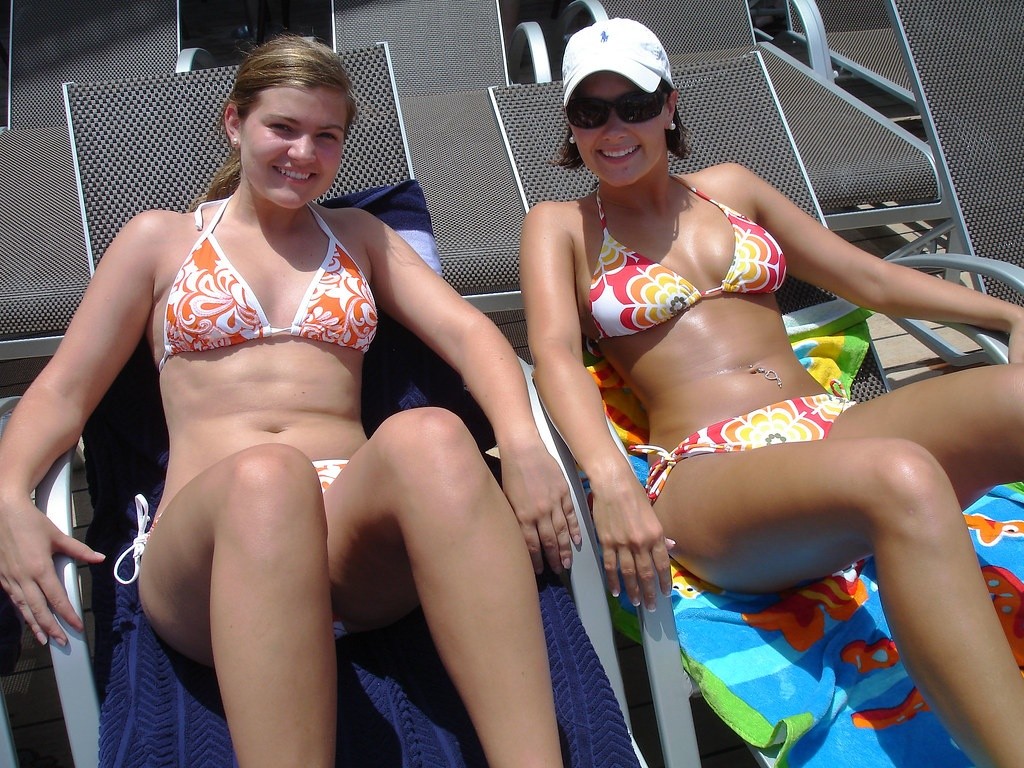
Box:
[0,41,645,768]
[473,50,1024,767]
[1,0,1024,362]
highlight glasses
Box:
[566,89,670,129]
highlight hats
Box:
[562,18,675,108]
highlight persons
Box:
[1,36,583,768]
[518,17,1024,766]
